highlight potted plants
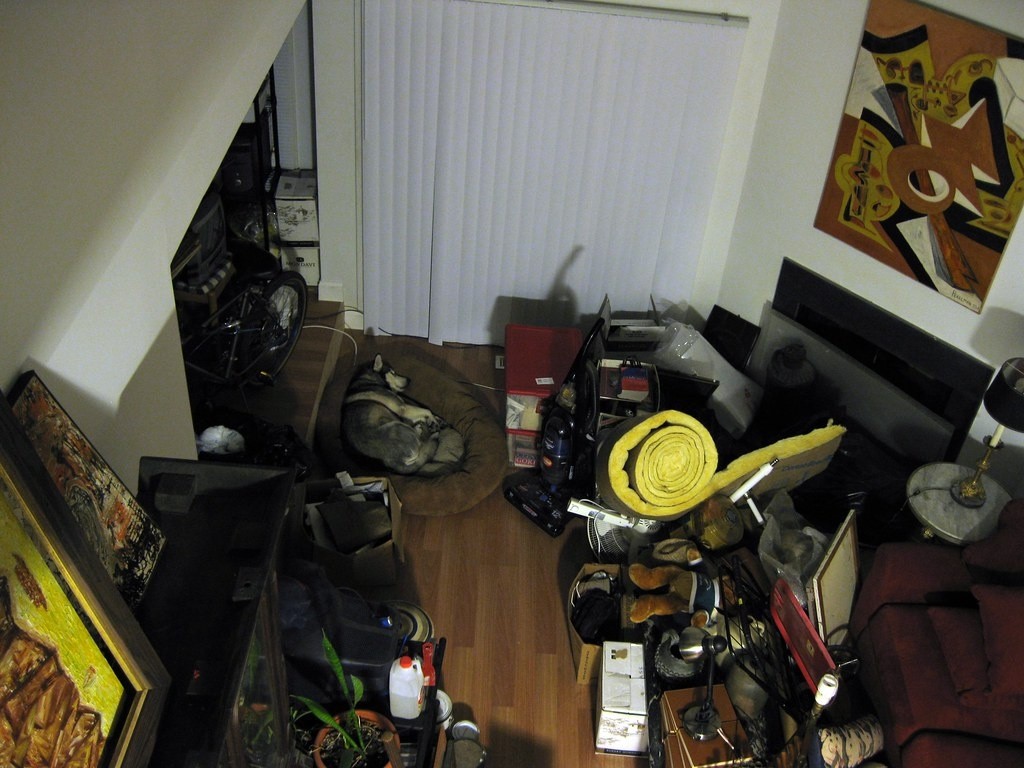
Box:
[291,629,401,768]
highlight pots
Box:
[434,690,453,732]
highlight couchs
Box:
[844,499,1024,768]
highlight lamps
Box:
[907,358,1024,545]
[678,627,728,740]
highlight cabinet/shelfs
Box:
[133,456,446,768]
[218,64,281,262]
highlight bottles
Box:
[390,656,424,719]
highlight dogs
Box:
[340,353,438,475]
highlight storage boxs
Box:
[304,476,405,573]
[275,176,320,288]
[591,295,666,364]
[566,562,633,685]
[595,642,656,757]
[505,322,586,467]
[716,546,771,600]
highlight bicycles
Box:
[182,270,308,408]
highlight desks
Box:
[174,256,237,329]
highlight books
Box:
[617,365,649,401]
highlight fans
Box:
[568,495,672,564]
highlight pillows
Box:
[818,714,885,768]
[926,583,1024,693]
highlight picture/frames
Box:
[767,579,836,698]
[807,509,862,654]
[0,391,174,768]
